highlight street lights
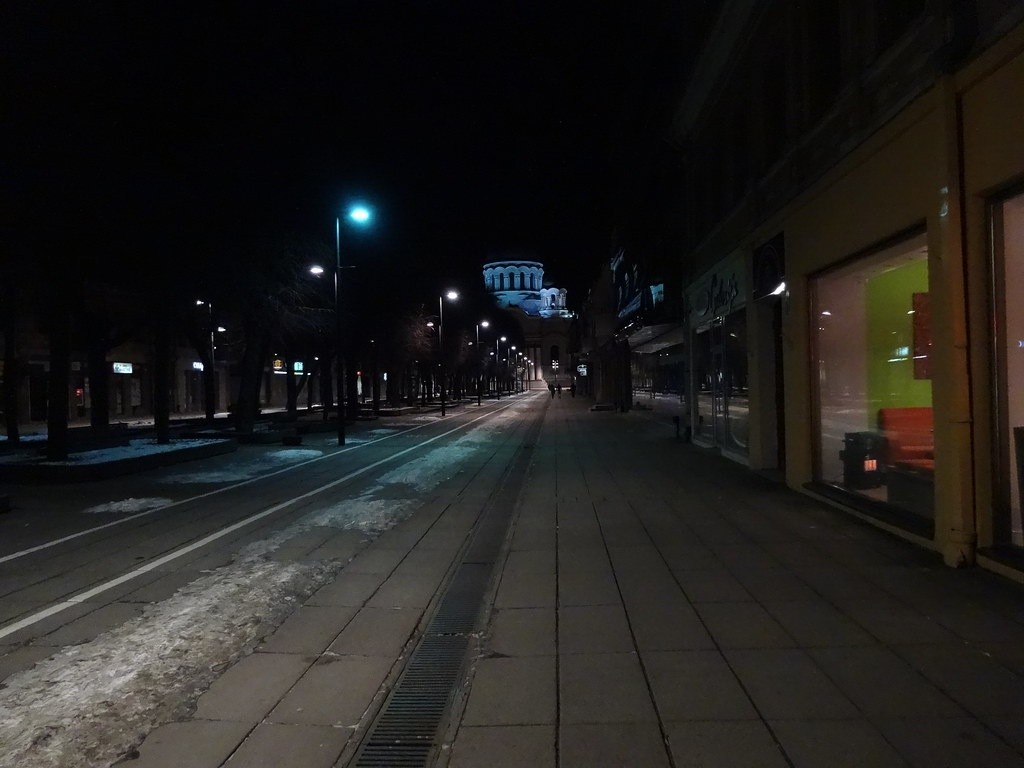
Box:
[495,334,506,400]
[474,318,489,404]
[551,359,559,381]
[505,344,516,397]
[514,352,534,394]
[195,300,227,386]
[332,200,377,444]
[439,287,457,411]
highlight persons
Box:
[547,383,551,391]
[555,383,562,399]
[550,384,555,399]
[570,382,576,397]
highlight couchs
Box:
[878,407,934,473]
